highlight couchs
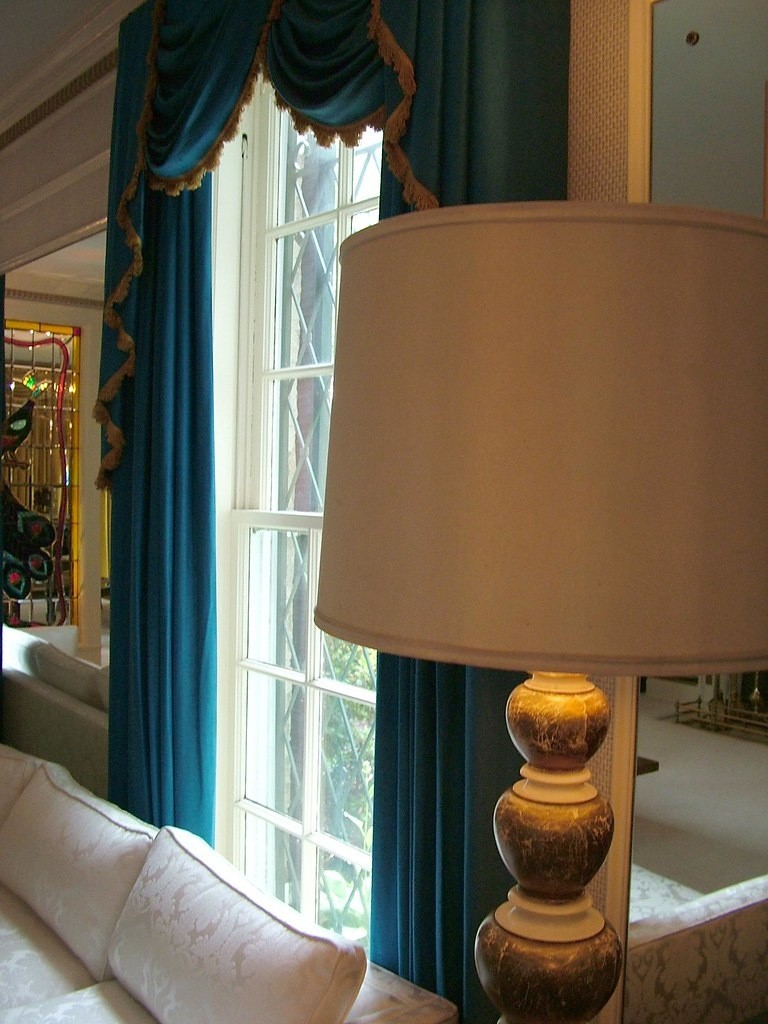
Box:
[0,881,458,1024]
[621,863,768,1024]
[1,623,109,801]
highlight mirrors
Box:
[619,669,768,1024]
[0,219,112,800]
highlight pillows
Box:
[0,744,45,830]
[107,826,367,1024]
[0,763,161,983]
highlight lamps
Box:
[313,204,768,1024]
